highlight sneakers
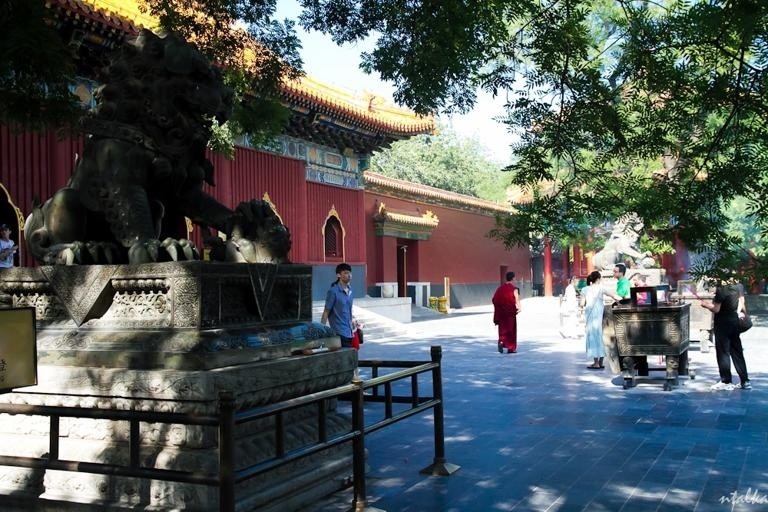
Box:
[497,343,517,353]
[712,381,752,391]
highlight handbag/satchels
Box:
[357,329,364,343]
[737,312,752,332]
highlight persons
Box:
[559,263,649,369]
[0,224,19,272]
[321,263,360,349]
[492,272,520,353]
[696,275,752,390]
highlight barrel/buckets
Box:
[429,297,438,309]
[438,296,448,311]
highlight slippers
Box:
[588,363,605,369]
